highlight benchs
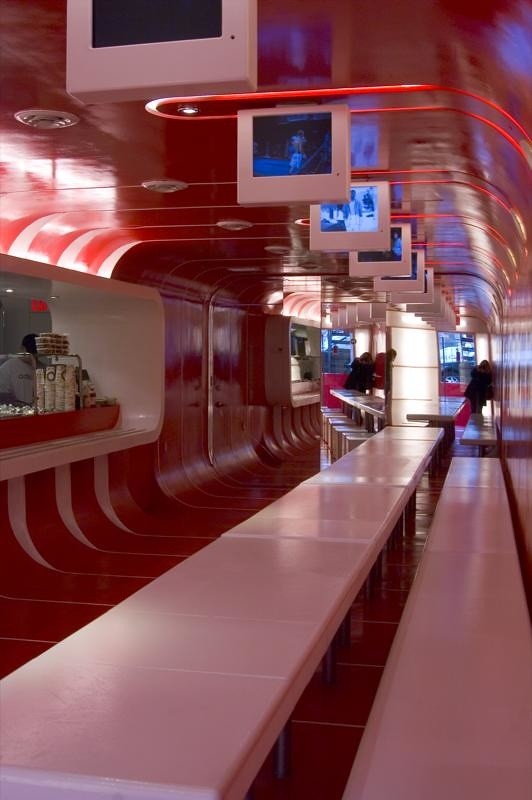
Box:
[343,457,531,800]
[460,413,497,458]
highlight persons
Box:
[341,190,362,232]
[343,352,374,393]
[0,333,40,405]
[374,349,396,390]
[289,130,307,175]
[391,232,402,261]
[463,360,494,415]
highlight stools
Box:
[320,407,370,461]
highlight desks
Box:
[345,397,381,422]
[360,402,385,433]
[407,396,466,445]
[330,389,365,415]
[0,426,446,800]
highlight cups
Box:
[64,366,75,411]
[88,383,96,408]
[35,369,45,409]
[55,365,64,410]
[45,367,55,411]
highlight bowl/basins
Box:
[35,333,71,354]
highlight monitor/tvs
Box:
[66,0,259,108]
[234,107,457,332]
